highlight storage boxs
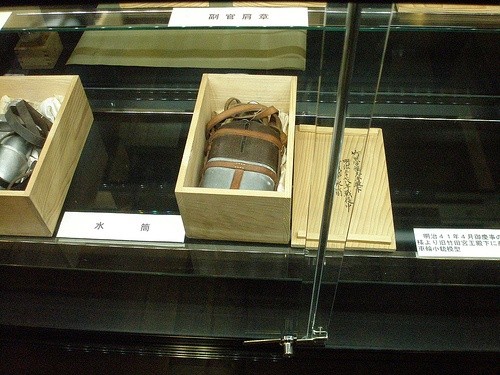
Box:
[14,31,62,68]
[174,73,297,244]
[0,75,93,237]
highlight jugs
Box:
[0,100,52,193]
[200,97,288,196]
